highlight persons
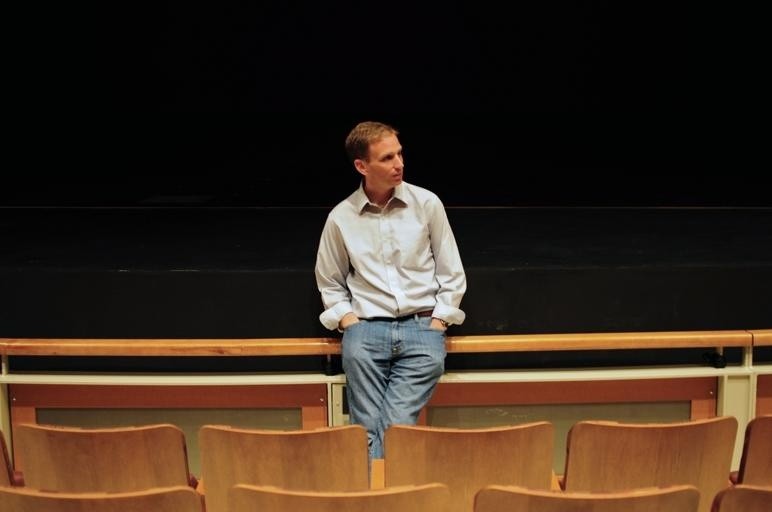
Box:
[313,120,469,459]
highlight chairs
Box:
[1,415,772,512]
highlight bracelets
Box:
[440,318,450,328]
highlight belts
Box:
[369,310,433,322]
